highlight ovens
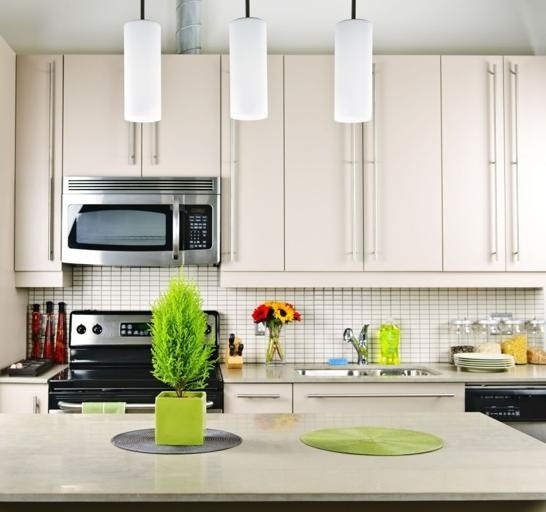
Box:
[50,393,223,414]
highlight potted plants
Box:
[143,266,222,446]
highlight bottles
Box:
[449,312,545,364]
[30,300,69,364]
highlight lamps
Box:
[334,0,372,123]
[229,0,267,122]
[123,1,163,126]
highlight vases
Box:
[265,322,286,365]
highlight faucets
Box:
[341,323,371,364]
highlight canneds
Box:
[450,318,546,366]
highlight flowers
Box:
[251,298,301,364]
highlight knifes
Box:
[228,333,244,356]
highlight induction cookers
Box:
[48,364,223,388]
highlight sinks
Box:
[291,365,444,379]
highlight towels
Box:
[82,402,128,415]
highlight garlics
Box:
[10,362,23,369]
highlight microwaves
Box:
[60,176,221,268]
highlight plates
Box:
[453,352,515,371]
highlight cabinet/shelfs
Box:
[220,54,282,288]
[16,52,73,289]
[439,54,545,288]
[223,381,293,415]
[1,378,49,416]
[61,53,220,179]
[283,52,439,290]
[293,382,465,416]
